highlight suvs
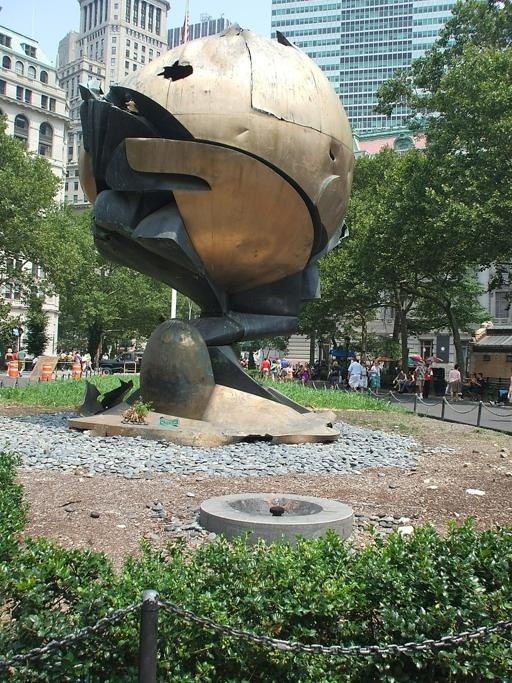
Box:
[100,352,144,376]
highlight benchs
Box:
[481,377,512,394]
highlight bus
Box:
[238,342,263,366]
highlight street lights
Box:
[18,327,23,351]
[345,336,350,367]
[317,340,323,363]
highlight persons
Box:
[239,356,248,370]
[60,350,109,373]
[392,354,512,407]
[4,347,27,377]
[259,357,383,395]
